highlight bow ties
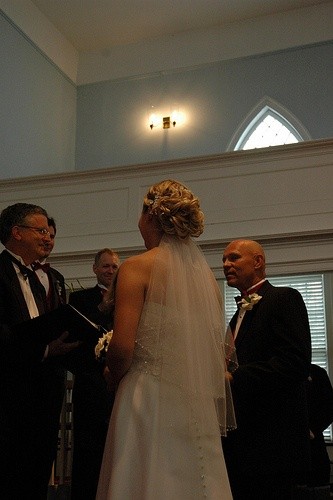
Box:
[98,286,107,295]
[9,254,33,277]
[236,282,263,306]
[31,261,49,273]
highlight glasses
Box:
[17,225,50,236]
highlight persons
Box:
[101,179,239,500]
[220,240,315,500]
[309,362,333,489]
[68,248,123,500]
[0,203,83,500]
[29,215,69,500]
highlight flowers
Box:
[237,290,262,310]
[95,330,114,361]
